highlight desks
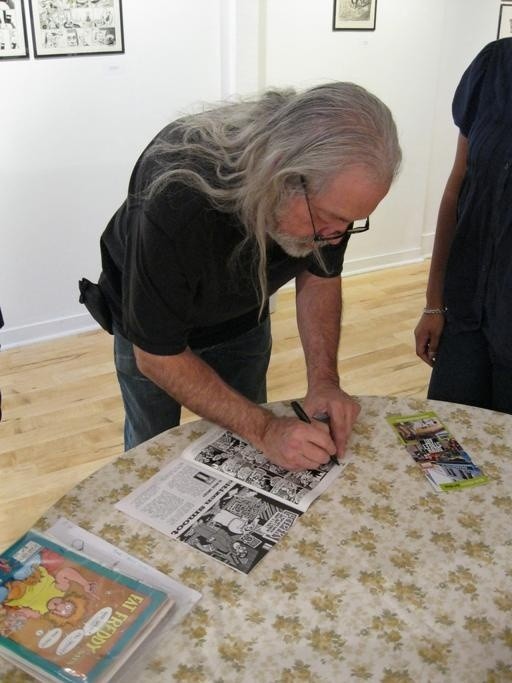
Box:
[0,393,510,682]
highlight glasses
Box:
[300,173,369,241]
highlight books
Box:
[0,515,206,682]
[115,419,356,572]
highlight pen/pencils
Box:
[291,401,341,467]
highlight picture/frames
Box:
[332,0,378,30]
[494,3,511,42]
[0,0,30,61]
[28,0,126,57]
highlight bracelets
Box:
[423,305,448,313]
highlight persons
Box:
[98,79,403,474]
[412,35,511,415]
